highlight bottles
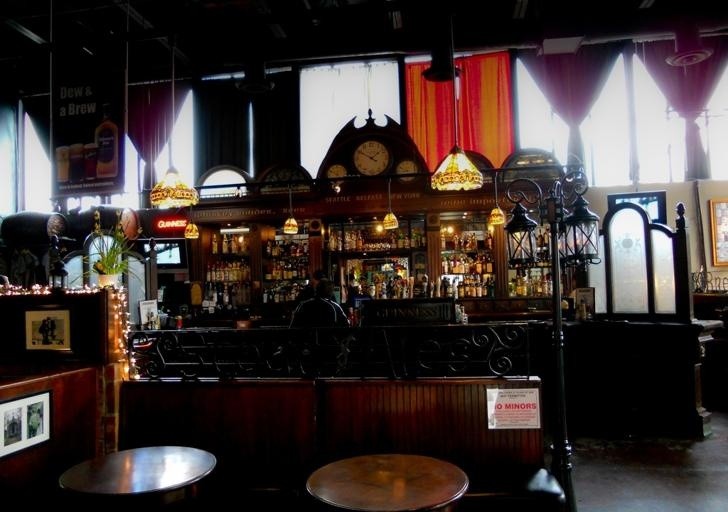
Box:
[204,226,553,306]
[459,307,470,325]
[347,305,358,327]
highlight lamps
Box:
[423,2,486,196]
[485,169,508,228]
[145,37,201,210]
[181,202,202,243]
[283,186,300,239]
[380,179,402,233]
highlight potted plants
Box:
[66,221,144,288]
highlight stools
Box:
[305,451,473,512]
[59,441,218,512]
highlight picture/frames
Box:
[708,197,728,266]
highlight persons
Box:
[27,406,41,438]
[288,269,350,328]
[39,316,56,345]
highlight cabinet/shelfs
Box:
[182,180,560,319]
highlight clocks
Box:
[325,141,420,183]
